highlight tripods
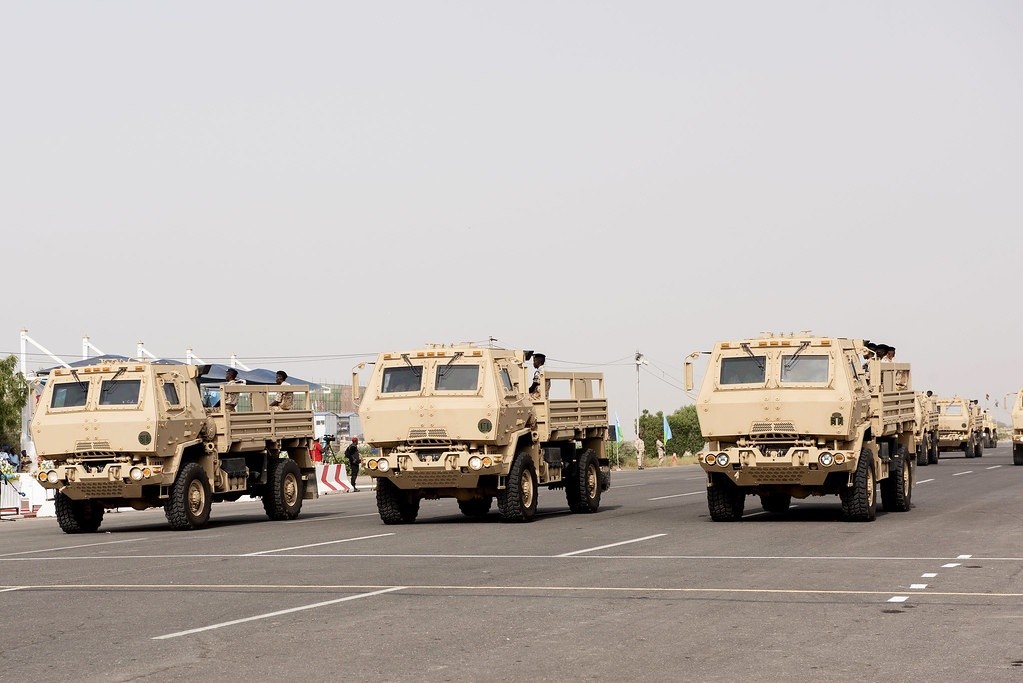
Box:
[323,441,339,464]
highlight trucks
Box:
[1003,388,1023,466]
[937,395,999,457]
[350,339,617,526]
[684,330,938,523]
[27,357,319,530]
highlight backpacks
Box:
[345,444,349,458]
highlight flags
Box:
[663,416,673,444]
[615,411,625,443]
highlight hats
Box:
[532,354,545,357]
[226,368,238,374]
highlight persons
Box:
[393,372,421,391]
[269,371,293,411]
[725,360,759,383]
[528,353,551,400]
[973,399,978,406]
[213,368,239,412]
[861,343,895,385]
[925,390,933,399]
[0,443,32,474]
[656,434,667,468]
[310,435,324,461]
[345,437,361,492]
[634,432,647,470]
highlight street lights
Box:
[635,351,644,440]
